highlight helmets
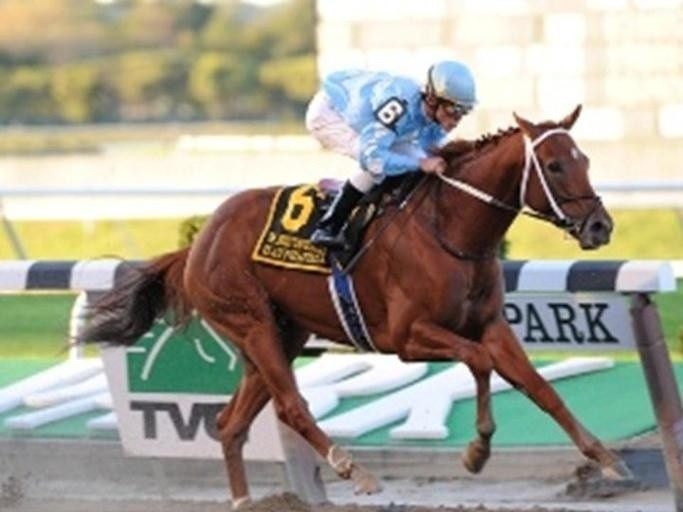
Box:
[419,59,479,108]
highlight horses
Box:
[53,103,635,509]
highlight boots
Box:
[310,180,366,249]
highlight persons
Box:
[304,59,479,253]
